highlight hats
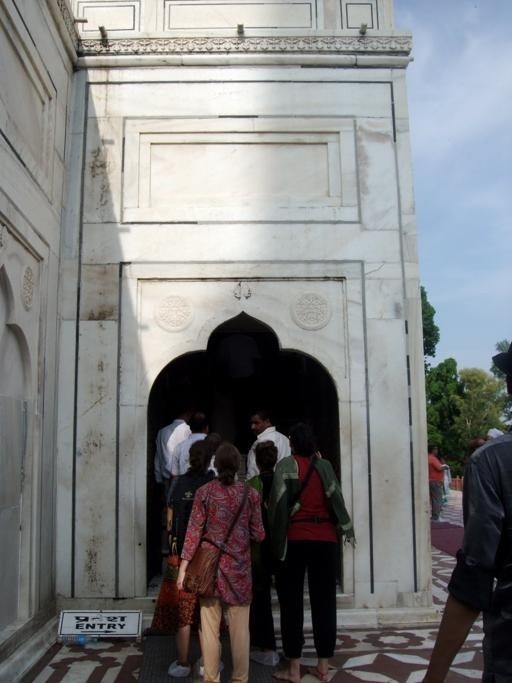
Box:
[492,342,512,376]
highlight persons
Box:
[177,444,265,682]
[204,433,239,480]
[169,411,209,479]
[166,440,225,677]
[246,409,292,480]
[268,422,356,682]
[246,441,303,664]
[428,441,451,522]
[154,409,192,497]
[419,342,511,682]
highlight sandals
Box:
[308,667,329,681]
[272,670,294,682]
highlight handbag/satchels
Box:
[166,542,179,593]
[184,541,221,597]
